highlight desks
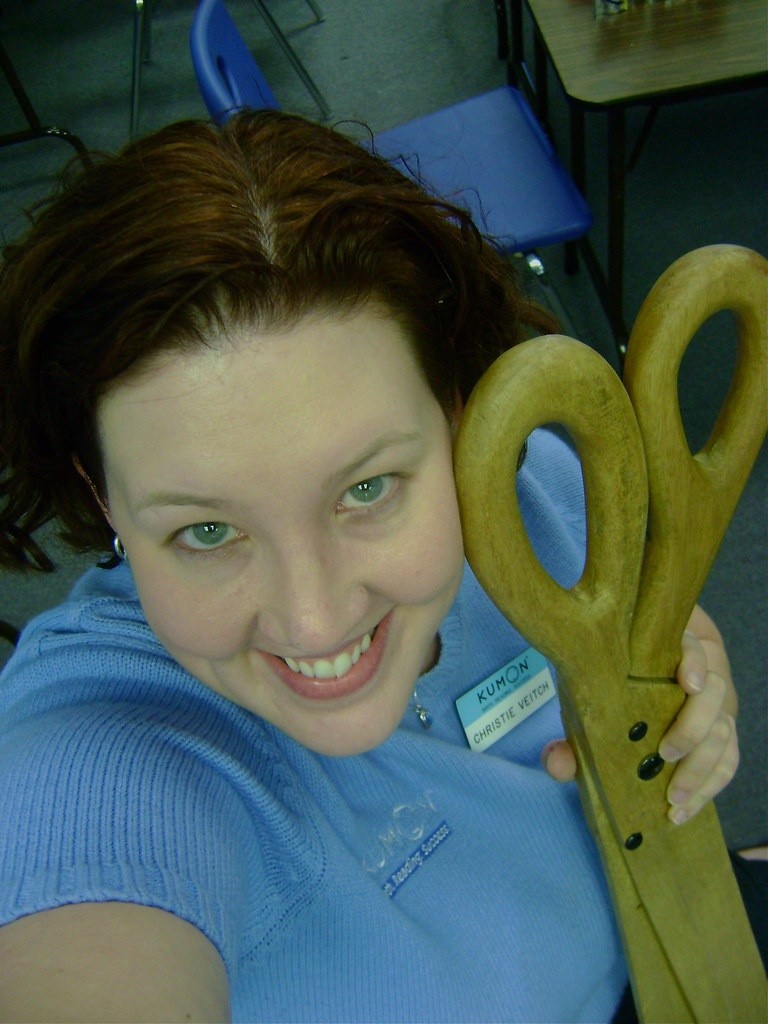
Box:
[527,1,768,380]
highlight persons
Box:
[0,110,740,1023]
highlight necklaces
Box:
[408,690,433,730]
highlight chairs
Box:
[191,1,595,346]
